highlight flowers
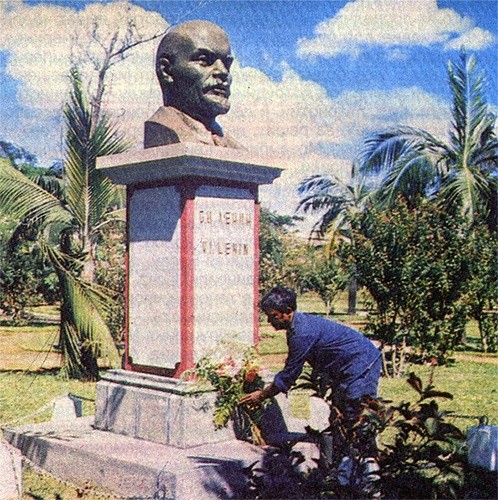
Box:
[176,332,273,447]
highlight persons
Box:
[238,283,383,467]
[143,18,257,151]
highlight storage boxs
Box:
[467,426,498,471]
